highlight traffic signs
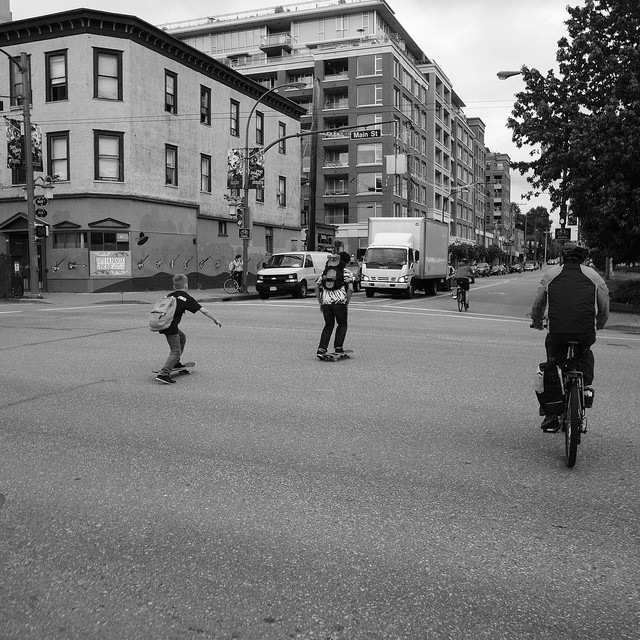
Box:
[350,129,382,140]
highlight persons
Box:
[529,240,610,428]
[155,274,223,384]
[225,254,244,289]
[457,258,475,308]
[314,251,354,360]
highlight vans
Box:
[256,251,333,299]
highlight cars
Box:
[469,265,478,278]
[489,265,502,276]
[524,263,535,272]
[509,264,523,273]
[437,266,458,291]
[547,258,555,265]
[500,264,507,275]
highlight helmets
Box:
[560,242,589,267]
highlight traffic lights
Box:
[559,204,567,224]
[36,226,46,237]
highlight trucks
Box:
[361,216,448,299]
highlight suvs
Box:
[477,263,491,277]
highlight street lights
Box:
[241,81,307,292]
[442,189,470,223]
[496,71,568,266]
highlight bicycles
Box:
[457,281,475,312]
[223,269,240,293]
[530,324,604,467]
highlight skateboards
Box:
[152,361,198,375]
[322,350,354,362]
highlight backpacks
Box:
[148,297,176,332]
[322,258,345,291]
[534,358,565,416]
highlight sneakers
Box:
[172,363,186,370]
[541,417,559,429]
[583,388,593,406]
[335,347,346,355]
[317,348,327,360]
[155,372,176,385]
